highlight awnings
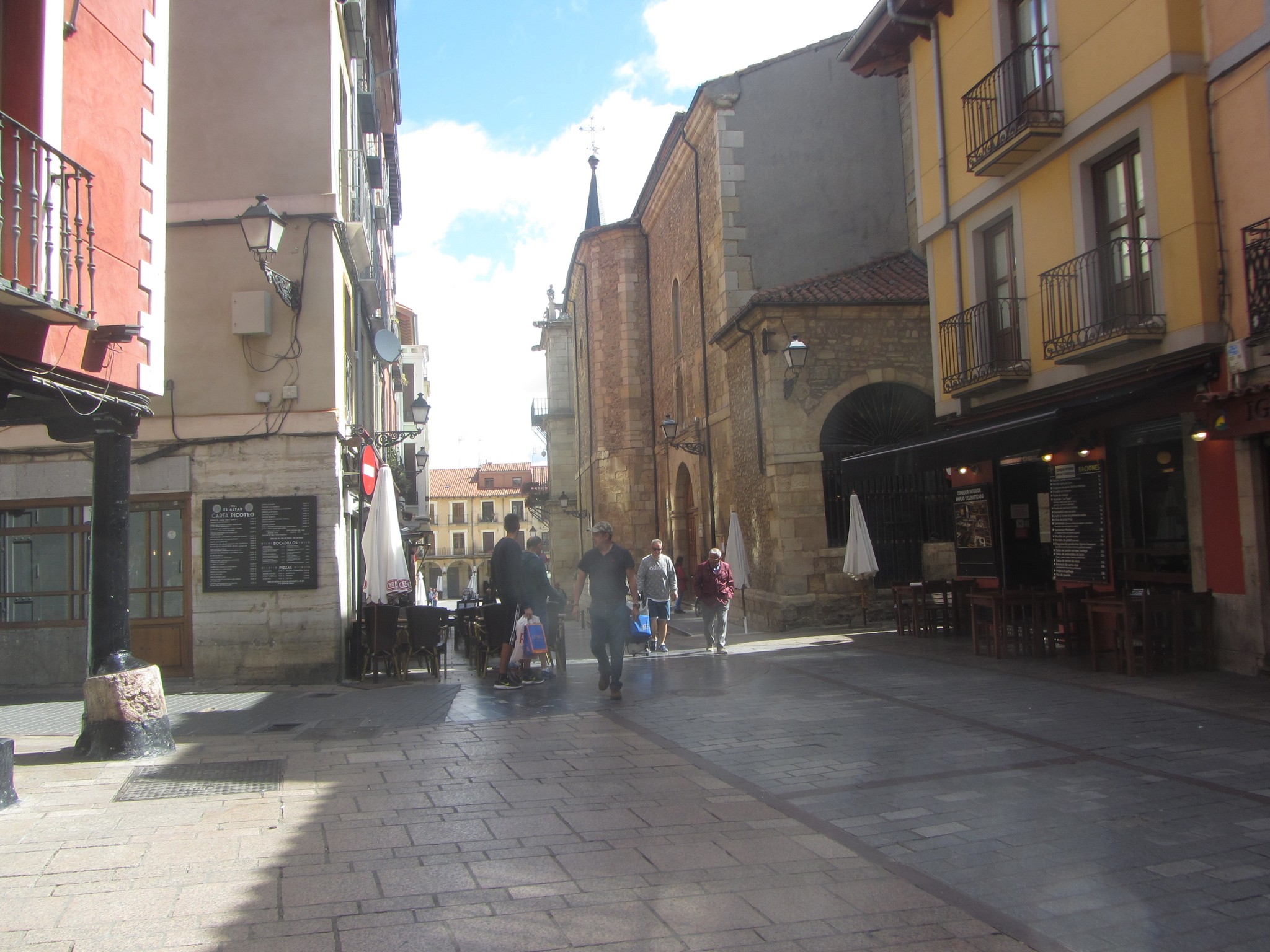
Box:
[840,360,1221,485]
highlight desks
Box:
[892,584,951,637]
[1081,596,1143,675]
[964,591,1059,659]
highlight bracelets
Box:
[572,604,579,608]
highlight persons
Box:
[573,522,639,700]
[427,587,434,605]
[490,513,544,689]
[432,589,438,607]
[554,583,568,622]
[636,539,678,652]
[509,536,571,683]
[693,548,735,654]
[482,588,497,605]
[673,557,687,613]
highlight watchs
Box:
[632,603,640,609]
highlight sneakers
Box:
[650,637,658,651]
[493,676,523,690]
[542,670,556,679]
[659,645,668,652]
[521,672,545,684]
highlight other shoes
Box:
[609,683,622,700]
[599,673,610,691]
[718,648,728,654]
[707,644,714,652]
[674,609,686,614]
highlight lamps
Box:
[530,525,549,545]
[403,446,429,480]
[1188,419,1206,442]
[1036,446,1055,461]
[958,464,979,475]
[784,334,808,398]
[458,562,462,568]
[92,324,144,344]
[442,567,447,574]
[236,194,300,313]
[660,415,706,456]
[375,393,430,448]
[1074,436,1095,457]
[428,563,432,569]
[472,566,478,573]
[558,491,587,519]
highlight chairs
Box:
[892,575,1213,677]
[363,601,565,683]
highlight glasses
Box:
[652,547,662,551]
[708,557,720,562]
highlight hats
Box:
[587,521,613,535]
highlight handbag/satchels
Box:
[510,614,548,663]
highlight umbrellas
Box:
[467,576,477,598]
[360,463,412,684]
[843,489,879,626]
[724,510,752,633]
[416,572,426,605]
